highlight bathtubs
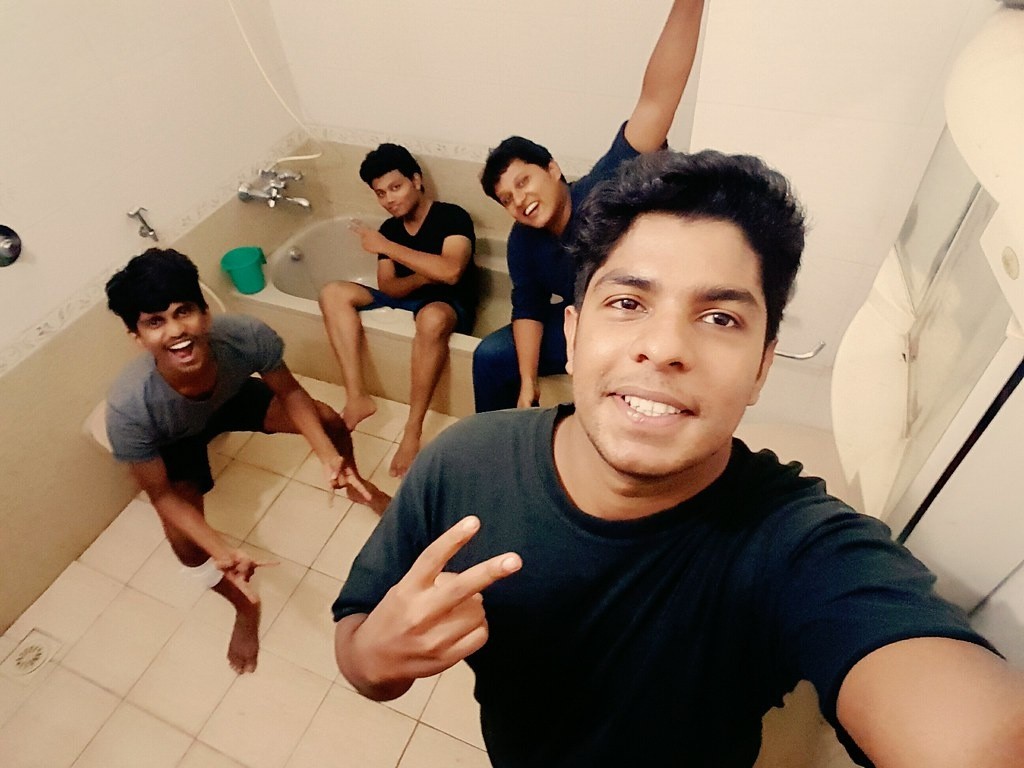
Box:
[230,200,575,421]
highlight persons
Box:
[332,149,1024,767]
[471,0,704,414]
[106,248,391,674]
[318,144,482,478]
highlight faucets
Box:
[238,168,312,214]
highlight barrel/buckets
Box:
[221,246,268,295]
